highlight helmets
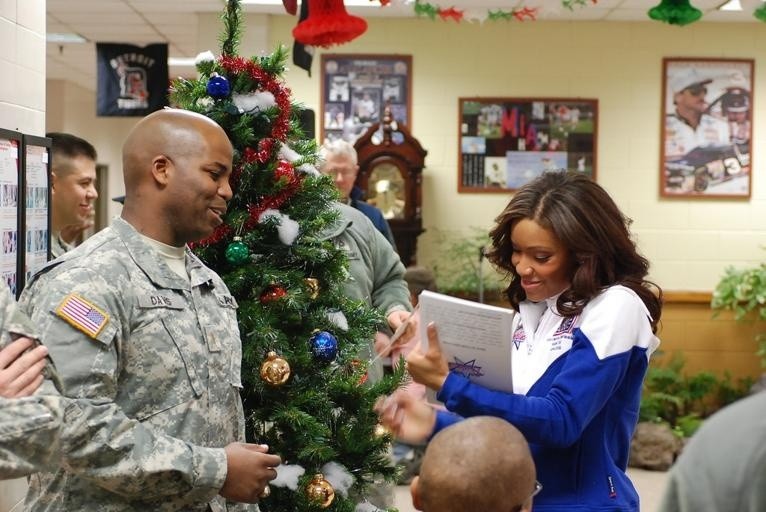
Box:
[722,93,750,113]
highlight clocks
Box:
[353,99,428,232]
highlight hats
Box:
[673,68,712,95]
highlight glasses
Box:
[691,84,708,96]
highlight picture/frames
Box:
[319,53,412,146]
[457,96,600,194]
[0,128,58,301]
[659,57,755,201]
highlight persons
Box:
[664,67,731,195]
[18,107,281,512]
[0,276,64,478]
[722,93,751,181]
[369,170,660,512]
[301,202,415,510]
[317,138,399,254]
[409,415,537,512]
[659,389,766,512]
[127,72,145,98]
[321,71,401,146]
[46,132,99,262]
